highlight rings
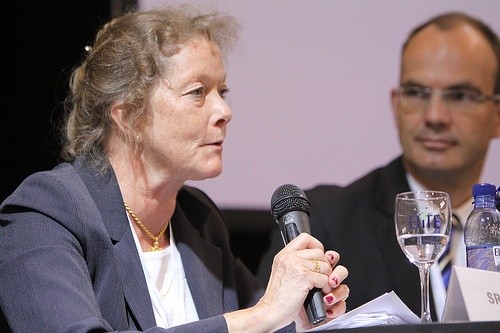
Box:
[311,258,321,274]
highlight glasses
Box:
[395,86,500,113]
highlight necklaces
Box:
[122,198,169,252]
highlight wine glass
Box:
[397,190,451,323]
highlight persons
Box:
[0,4,351,333]
[271,11,500,322]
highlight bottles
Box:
[462,183,499,272]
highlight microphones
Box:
[271,183,327,327]
